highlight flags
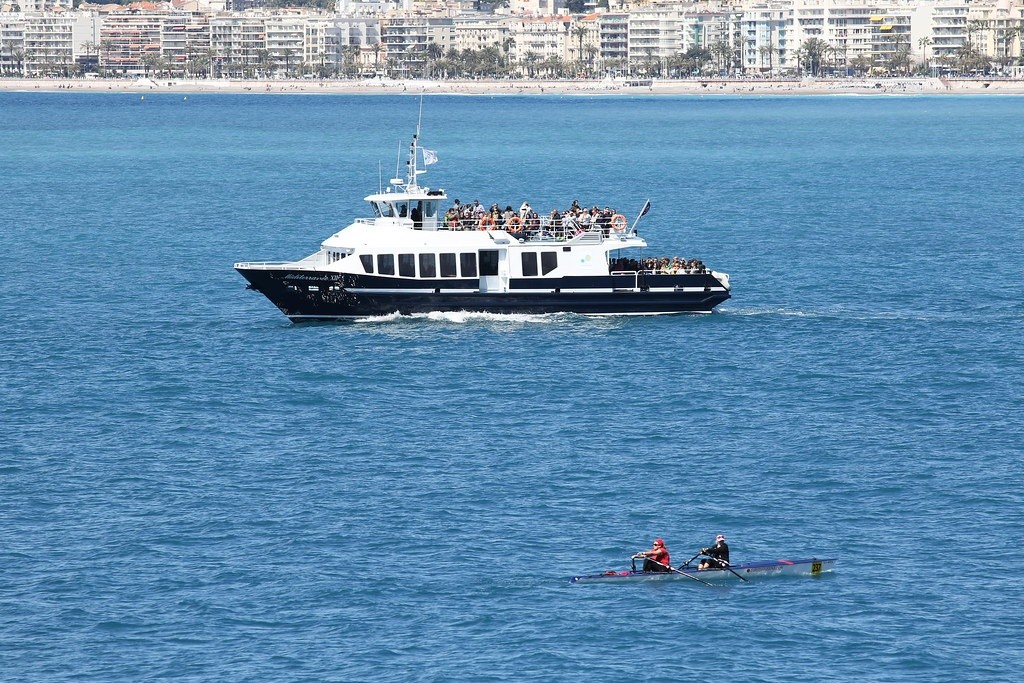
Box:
[641,201,650,216]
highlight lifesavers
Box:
[480,217,496,231]
[507,217,522,233]
[610,213,628,230]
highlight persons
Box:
[423,149,438,166]
[551,200,616,239]
[698,535,729,571]
[636,539,670,572]
[610,256,706,274]
[399,204,407,216]
[444,199,540,235]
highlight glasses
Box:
[653,544,659,546]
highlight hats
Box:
[583,209,588,211]
[717,535,725,542]
[656,539,663,548]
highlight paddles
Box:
[677,547,749,582]
[631,558,636,571]
[641,554,714,587]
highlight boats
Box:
[571,557,839,581]
[234,91,731,324]
